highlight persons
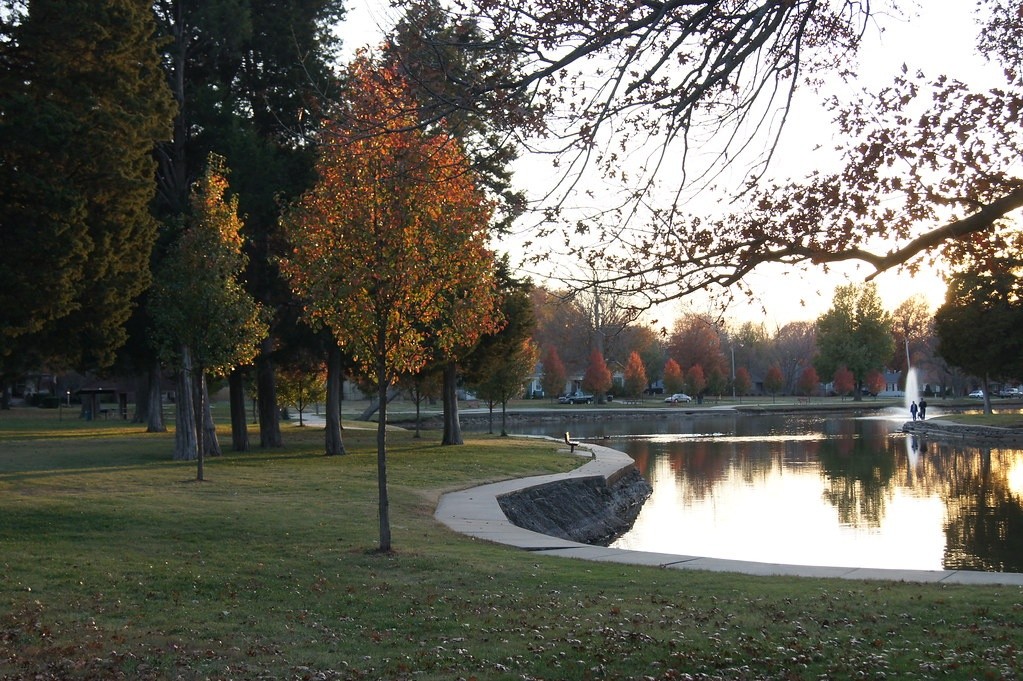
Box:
[911,401,918,420]
[919,398,927,419]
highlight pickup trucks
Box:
[558,392,593,404]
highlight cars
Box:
[969,390,984,399]
[665,394,692,403]
[1000,388,1023,399]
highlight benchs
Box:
[623,399,646,405]
[466,400,480,408]
[564,431,579,454]
[798,397,810,406]
